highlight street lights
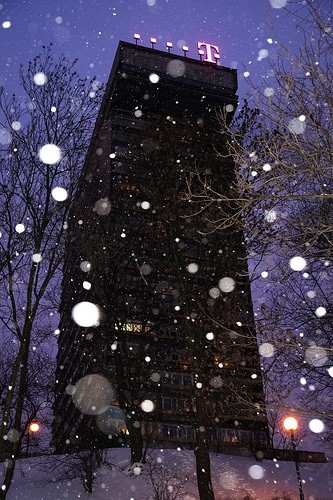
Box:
[26,419,38,457]
[285,417,305,500]
